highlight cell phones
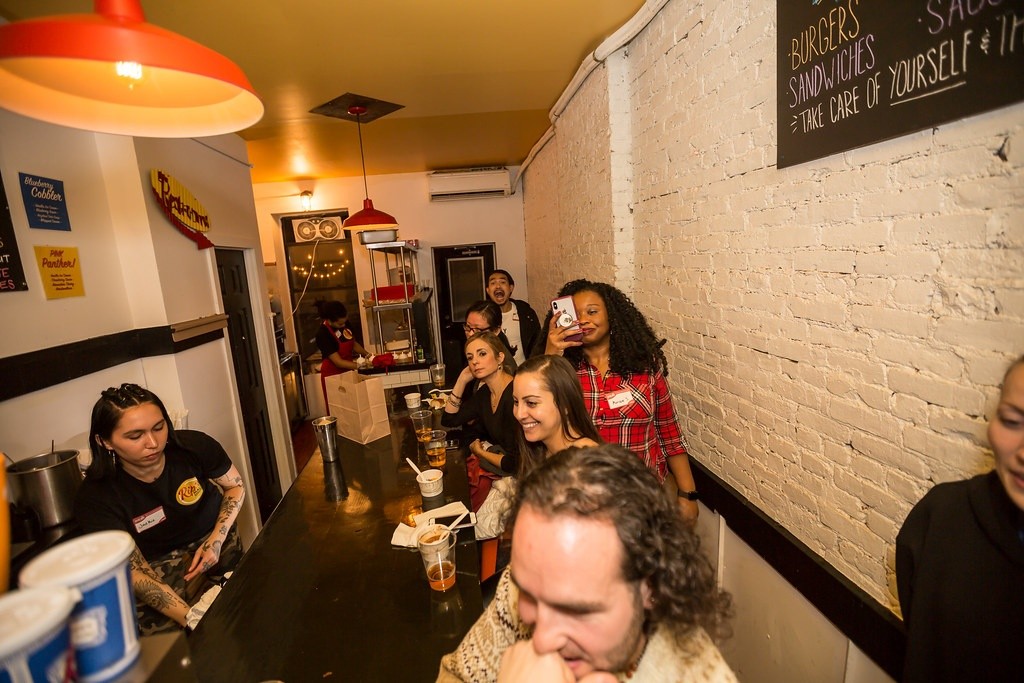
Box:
[549,296,583,342]
[429,512,477,529]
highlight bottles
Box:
[415,239,418,249]
[417,345,424,360]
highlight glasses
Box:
[463,322,492,333]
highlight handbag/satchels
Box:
[466,445,513,513]
[324,371,391,444]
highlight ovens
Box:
[279,352,310,439]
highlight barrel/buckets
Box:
[6,450,83,526]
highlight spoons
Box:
[432,511,468,543]
[429,389,452,394]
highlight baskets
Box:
[371,285,414,301]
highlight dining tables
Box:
[142,418,483,683]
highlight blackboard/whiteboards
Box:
[0,171,29,291]
[776,0,1024,169]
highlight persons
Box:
[541,278,699,526]
[513,355,602,473]
[316,301,378,416]
[485,269,541,367]
[465,300,518,356]
[436,444,739,683]
[80,382,246,670]
[895,356,1024,683]
[440,333,520,512]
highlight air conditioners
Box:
[426,165,512,203]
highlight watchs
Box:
[678,489,699,500]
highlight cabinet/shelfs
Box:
[363,241,419,367]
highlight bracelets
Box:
[448,392,462,407]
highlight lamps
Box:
[342,104,399,231]
[0,0,264,141]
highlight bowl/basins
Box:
[418,359,426,363]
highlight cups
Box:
[421,430,447,466]
[422,279,430,291]
[20,530,142,683]
[417,529,457,591]
[410,410,433,442]
[416,469,444,497]
[0,586,84,683]
[405,393,421,408]
[312,416,340,463]
[430,364,445,387]
[418,524,451,562]
